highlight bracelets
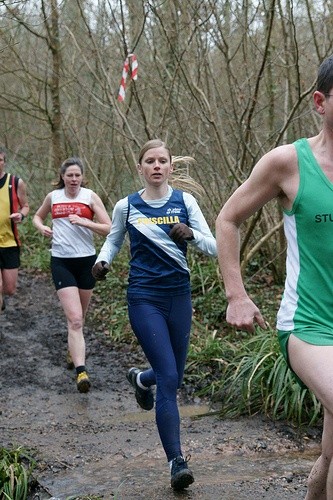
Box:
[20,213,25,221]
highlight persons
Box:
[216,55,332,500]
[0,148,29,311]
[92,140,218,491]
[33,159,112,393]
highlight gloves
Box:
[91,261,110,281]
[169,222,195,245]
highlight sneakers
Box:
[126,367,154,410]
[170,454,194,491]
[67,350,73,364]
[76,371,91,394]
